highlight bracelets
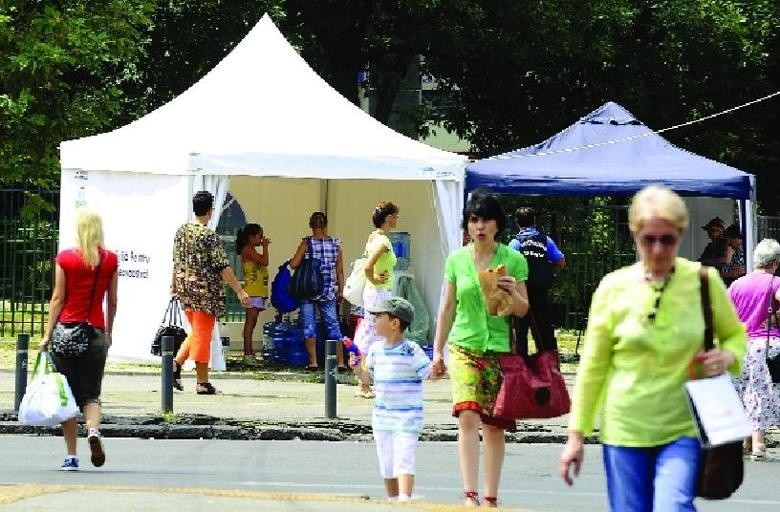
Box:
[170,284,176,288]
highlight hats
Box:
[700,217,726,232]
[370,297,413,324]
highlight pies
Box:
[484,263,506,276]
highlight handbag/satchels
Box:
[685,374,754,449]
[150,325,186,356]
[494,350,571,419]
[50,323,94,358]
[342,258,368,307]
[19,374,79,425]
[694,442,743,499]
[288,258,323,296]
[272,259,299,313]
[767,351,780,383]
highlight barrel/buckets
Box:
[262,315,323,367]
[422,344,434,361]
[388,232,411,271]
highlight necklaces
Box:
[643,265,674,320]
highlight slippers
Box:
[306,364,317,370]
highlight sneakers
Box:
[88,428,105,467]
[59,456,80,473]
[244,353,257,366]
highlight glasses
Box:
[638,234,678,245]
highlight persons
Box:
[235,223,273,367]
[355,201,401,398]
[36,211,119,472]
[349,296,448,501]
[168,189,251,396]
[559,186,748,512]
[700,215,780,459]
[508,207,567,355]
[432,192,533,509]
[289,212,349,372]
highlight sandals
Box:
[172,360,183,390]
[196,383,221,394]
